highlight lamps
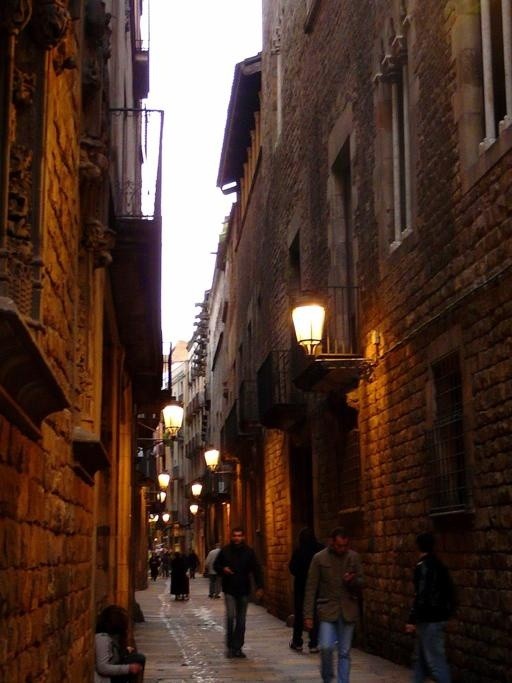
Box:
[290,302,380,382]
[133,404,183,439]
[204,449,237,480]
[148,468,171,490]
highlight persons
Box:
[213,527,256,660]
[147,542,199,581]
[204,542,222,599]
[94,605,144,682]
[169,552,190,601]
[288,527,326,653]
[404,531,458,683]
[302,529,370,683]
